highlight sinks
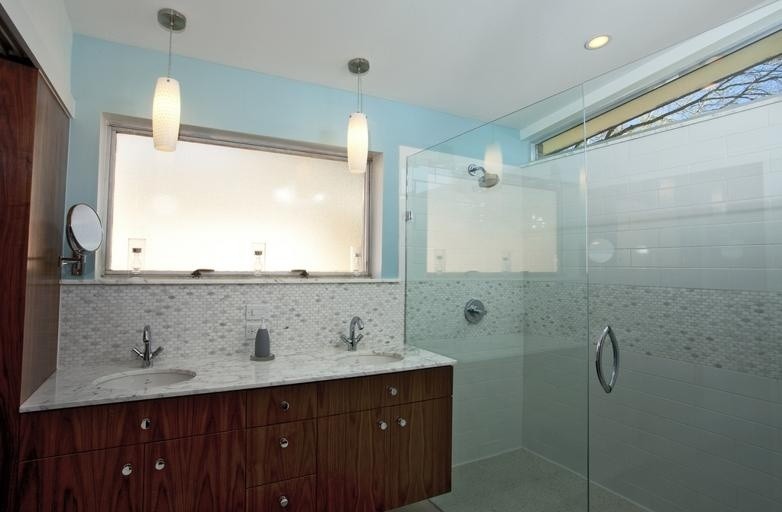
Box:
[332,350,404,367]
[93,369,197,392]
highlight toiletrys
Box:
[253,319,271,357]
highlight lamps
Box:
[348,57,369,174]
[152,8,187,152]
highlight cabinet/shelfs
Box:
[21,390,245,512]
[245,382,317,512]
[318,367,453,511]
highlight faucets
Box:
[131,324,164,368]
[341,316,364,351]
